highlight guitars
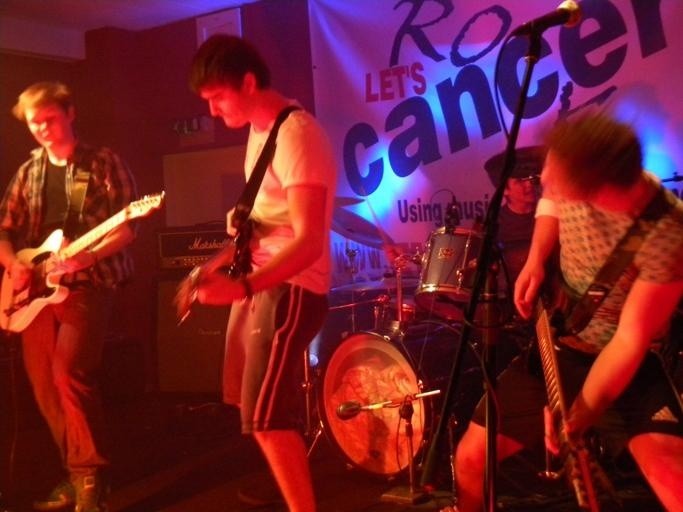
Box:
[177,222,254,326]
[510,266,603,512]
[0,191,165,333]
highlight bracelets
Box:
[239,274,251,297]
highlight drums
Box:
[330,210,393,247]
[415,226,503,322]
[323,320,488,474]
[388,299,435,337]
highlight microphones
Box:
[451,195,460,225]
[511,0,583,37]
[336,389,440,419]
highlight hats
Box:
[484,153,541,179]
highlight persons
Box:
[455,116,683,512]
[186,34,336,512]
[0,82,138,512]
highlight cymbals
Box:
[332,278,419,294]
[484,147,549,179]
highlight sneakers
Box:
[31,482,77,512]
[74,490,102,512]
